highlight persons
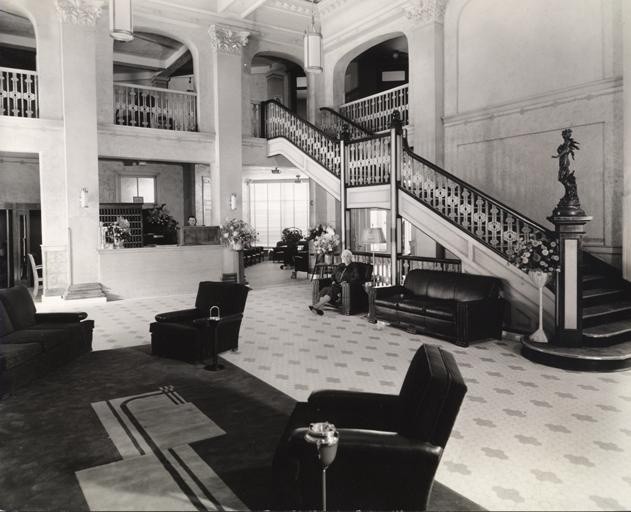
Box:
[309,250,353,316]
[187,215,197,226]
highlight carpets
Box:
[0,344,487,512]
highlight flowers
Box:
[282,227,303,242]
[222,218,256,250]
[505,238,562,273]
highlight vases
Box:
[287,240,298,255]
[528,273,551,344]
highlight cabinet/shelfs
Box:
[99,203,145,247]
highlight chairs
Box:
[148,281,251,364]
[272,241,287,262]
[312,263,373,316]
[273,344,467,511]
[243,245,265,267]
[117,93,155,127]
[28,253,43,296]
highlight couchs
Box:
[369,269,504,346]
[0,286,94,398]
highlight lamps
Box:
[110,0,134,42]
[80,188,88,208]
[303,0,323,73]
[231,193,238,209]
[359,224,386,276]
